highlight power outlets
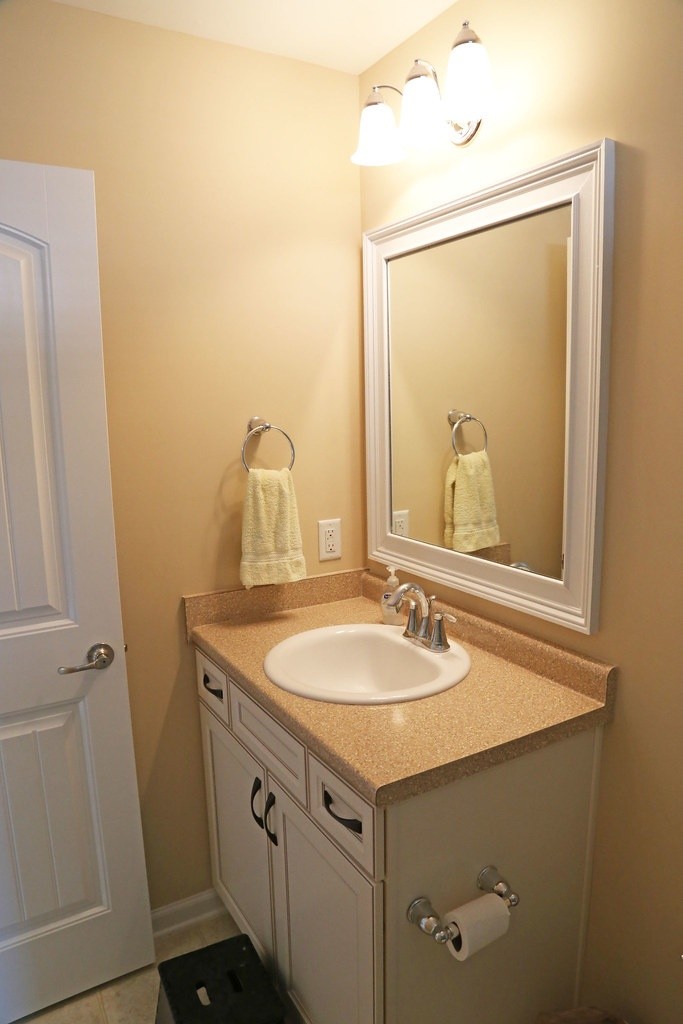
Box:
[392,509,409,535]
[317,518,343,562]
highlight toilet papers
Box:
[442,892,511,962]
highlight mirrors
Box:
[367,136,621,633]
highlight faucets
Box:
[388,583,432,639]
[509,561,531,572]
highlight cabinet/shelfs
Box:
[192,646,598,1024]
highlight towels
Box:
[444,451,500,553]
[241,469,307,589]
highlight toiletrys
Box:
[381,564,404,625]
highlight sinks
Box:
[265,623,472,705]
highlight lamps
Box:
[353,19,502,168]
